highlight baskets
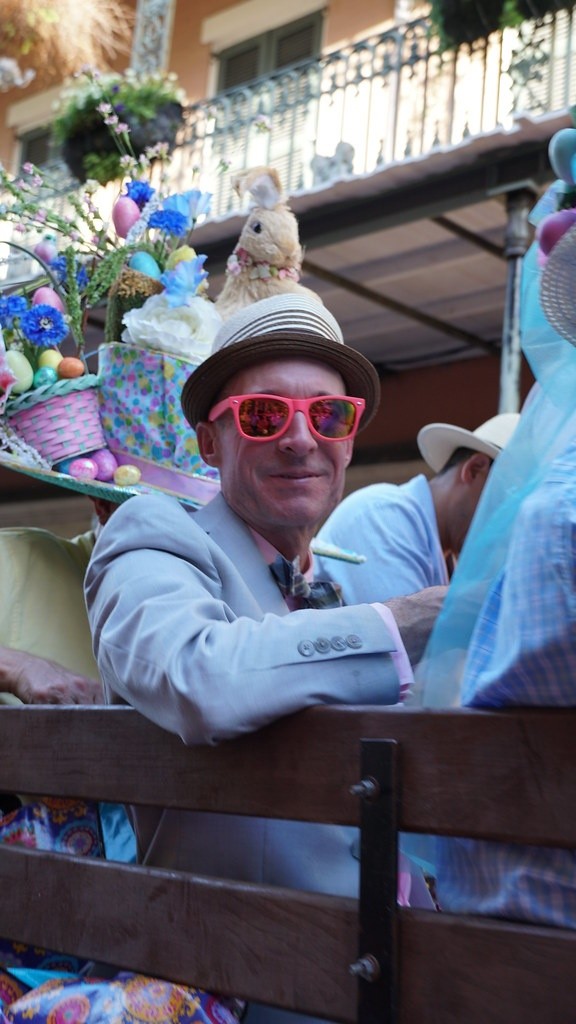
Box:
[5,374,108,467]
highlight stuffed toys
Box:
[215,168,325,315]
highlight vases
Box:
[61,102,182,181]
[441,0,576,42]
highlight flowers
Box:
[42,64,188,147]
[0,114,273,416]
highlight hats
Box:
[416,413,523,473]
[180,294,381,432]
[540,222,576,347]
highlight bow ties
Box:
[268,554,340,611]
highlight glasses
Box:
[206,393,366,442]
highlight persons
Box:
[433,214,576,932]
[83,292,503,1024]
[310,408,523,608]
[0,643,105,707]
